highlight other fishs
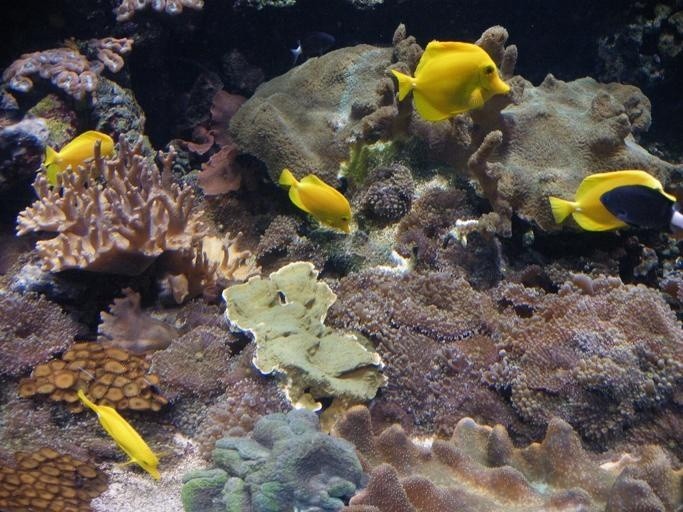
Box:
[390,41,511,121]
